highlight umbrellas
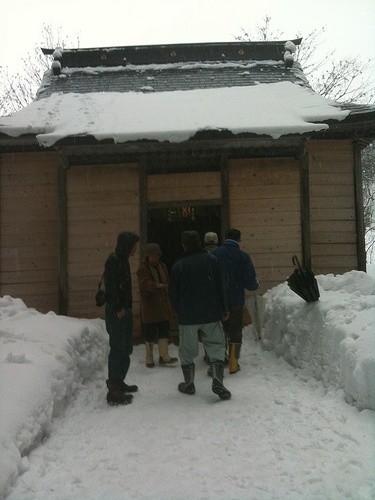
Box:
[287,255,320,303]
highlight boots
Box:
[178,363,195,395]
[229,342,240,374]
[210,361,231,400]
[158,338,178,364]
[207,362,224,379]
[145,342,155,368]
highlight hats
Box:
[181,231,201,252]
[204,232,219,245]
[142,244,163,262]
[115,231,140,259]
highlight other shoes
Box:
[107,384,138,392]
[106,390,134,405]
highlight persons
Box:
[204,232,229,376]
[103,232,139,403]
[212,229,259,373]
[136,243,178,367]
[169,231,231,399]
